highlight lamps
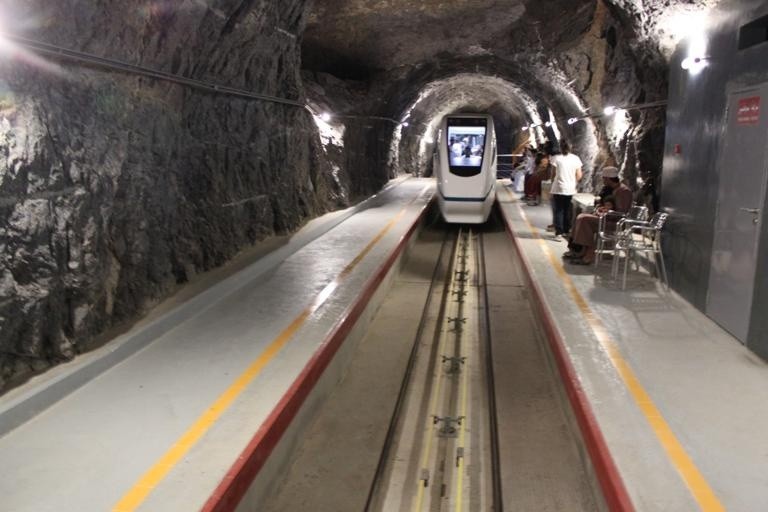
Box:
[681,53,713,72]
[520,102,636,132]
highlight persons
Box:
[449,134,483,166]
[510,136,634,266]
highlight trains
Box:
[431,113,499,226]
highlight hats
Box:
[602,167,618,178]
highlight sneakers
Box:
[565,249,594,266]
[521,194,540,206]
[546,224,568,242]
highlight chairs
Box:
[508,155,672,292]
